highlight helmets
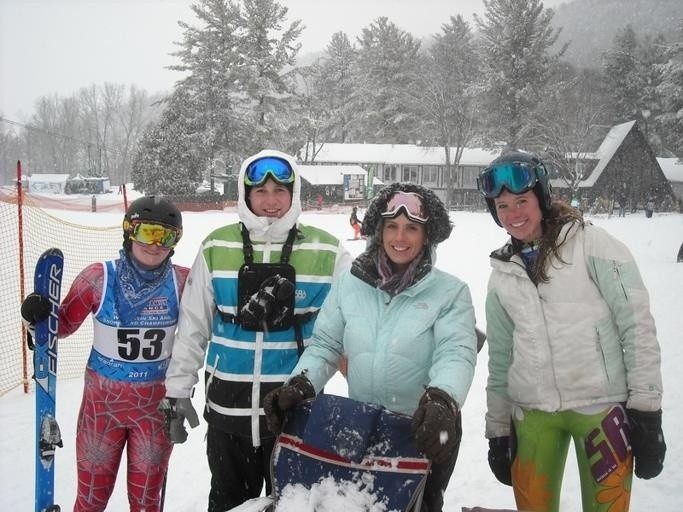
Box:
[124,195,182,241]
[484,151,550,227]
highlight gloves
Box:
[488,436,514,485]
[21,292,53,322]
[626,408,665,479]
[413,386,461,463]
[158,397,199,443]
[263,373,316,435]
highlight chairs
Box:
[218,394,433,511]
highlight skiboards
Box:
[33,248,64,511]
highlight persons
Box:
[20,195,192,512]
[579,193,655,218]
[480,152,666,512]
[165,150,354,512]
[350,207,363,239]
[266,179,478,512]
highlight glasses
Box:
[477,162,548,199]
[121,219,182,249]
[379,191,432,225]
[245,158,295,186]
